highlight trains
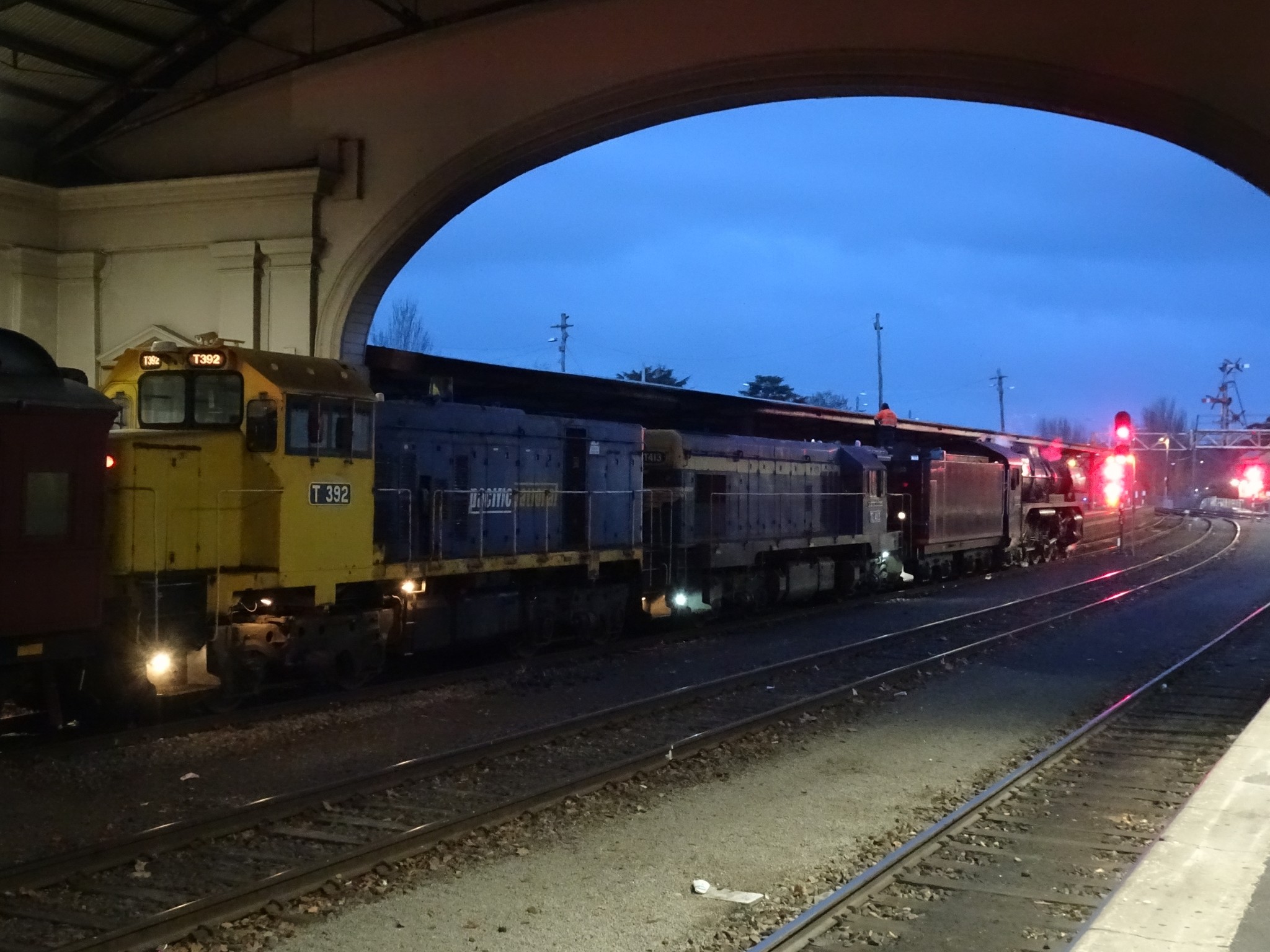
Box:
[0,314,1086,727]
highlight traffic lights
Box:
[1113,411,1133,455]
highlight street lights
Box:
[988,368,1015,433]
[546,313,575,372]
